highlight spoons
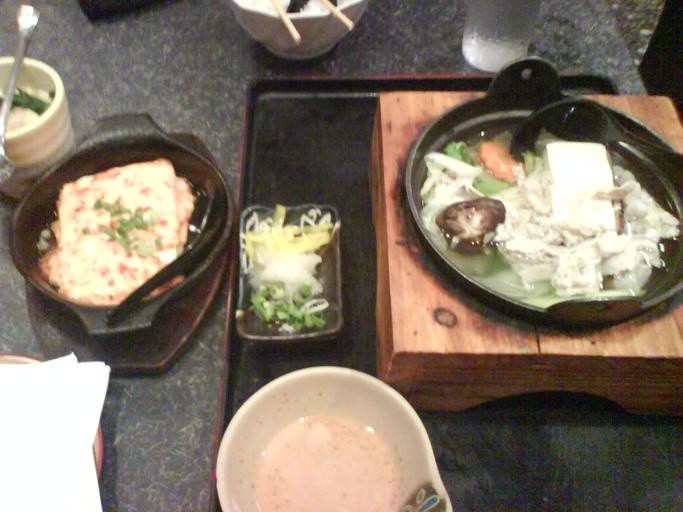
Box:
[105,175,230,324]
[513,99,683,168]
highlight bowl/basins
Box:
[215,363,454,511]
[229,1,369,62]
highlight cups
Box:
[1,57,75,202]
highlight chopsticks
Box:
[270,1,356,45]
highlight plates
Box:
[234,202,345,344]
